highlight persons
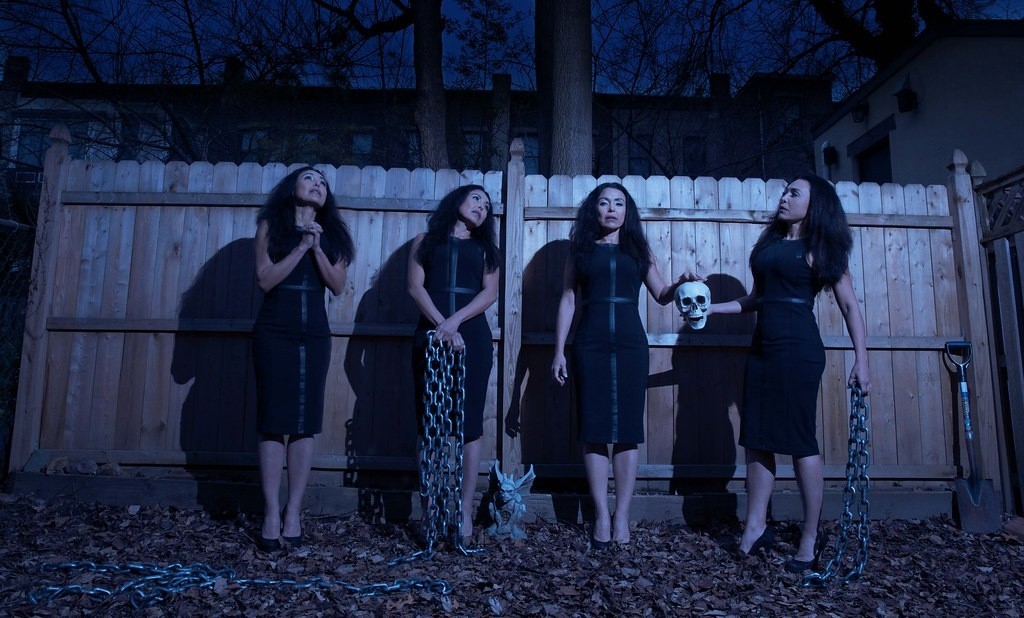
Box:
[407,184,500,550]
[548,180,708,549]
[252,166,355,552]
[681,174,870,575]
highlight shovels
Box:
[945,341,1002,535]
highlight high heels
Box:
[735,523,775,562]
[612,512,631,546]
[592,509,612,552]
[282,509,301,551]
[260,511,281,553]
[784,541,821,575]
